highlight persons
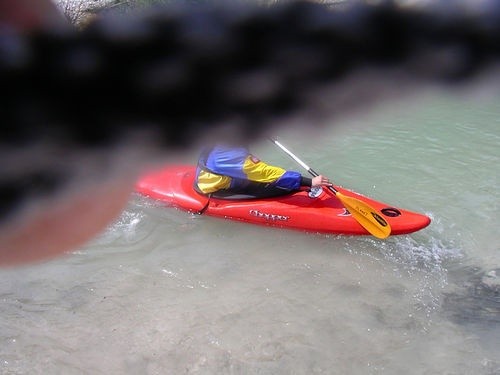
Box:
[193,142,333,199]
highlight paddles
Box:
[269,136,392,240]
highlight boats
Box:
[134,164,431,236]
